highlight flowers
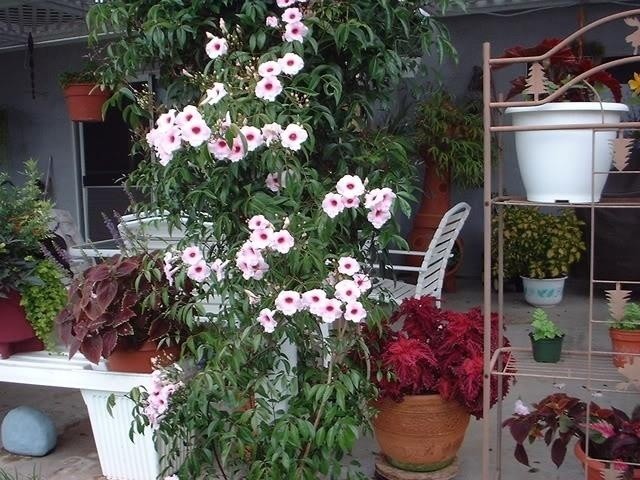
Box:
[56,181,178,364]
[115,0,424,480]
[502,391,640,480]
[622,16,640,98]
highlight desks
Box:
[68,238,299,434]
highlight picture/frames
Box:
[574,437,640,480]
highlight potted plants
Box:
[57,62,119,123]
[393,77,502,293]
[489,37,629,204]
[608,302,640,368]
[528,308,565,363]
[491,188,586,308]
[352,295,516,472]
[0,157,73,360]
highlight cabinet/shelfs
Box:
[482,7,640,480]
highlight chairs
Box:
[319,200,471,368]
[119,209,212,222]
[116,217,242,333]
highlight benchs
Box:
[0,345,195,480]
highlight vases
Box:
[107,341,182,373]
